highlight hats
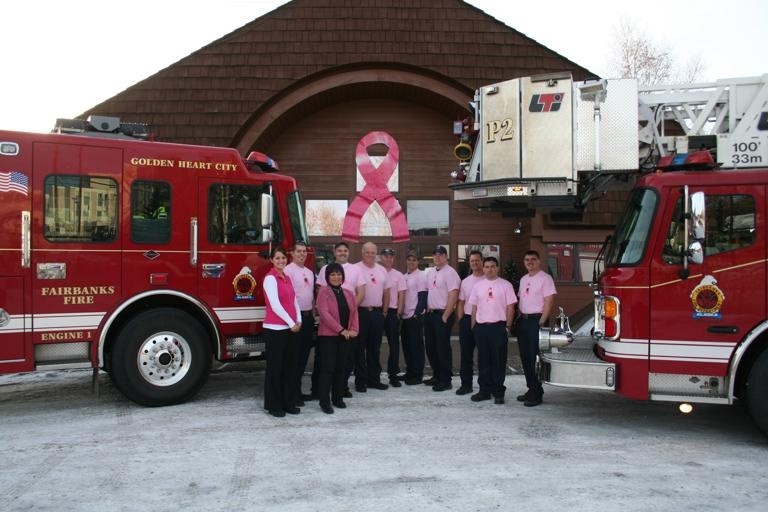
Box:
[381,248,395,255]
[432,246,447,254]
[406,251,417,256]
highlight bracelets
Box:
[506,325,511,328]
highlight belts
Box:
[360,306,382,312]
[428,309,438,313]
[519,313,540,319]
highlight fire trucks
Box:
[1,113,319,408]
[447,72,768,434]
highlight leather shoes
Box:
[343,388,352,398]
[355,383,366,392]
[269,394,313,417]
[517,387,543,407]
[471,387,504,404]
[423,376,452,391]
[456,385,473,395]
[367,383,388,390]
[389,373,423,387]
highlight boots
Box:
[322,405,334,414]
[333,395,345,408]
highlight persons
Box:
[424,246,461,391]
[316,262,360,414]
[379,248,407,387]
[285,241,312,407]
[262,247,299,418]
[456,250,484,395]
[354,242,390,392]
[514,251,559,406]
[471,256,518,405]
[313,242,366,398]
[401,252,428,385]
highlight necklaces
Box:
[334,287,342,294]
